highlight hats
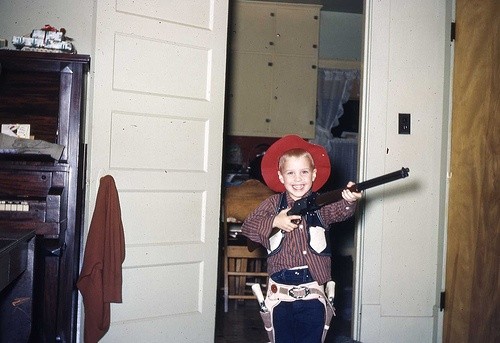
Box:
[261,134,331,192]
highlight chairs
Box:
[222,179,272,312]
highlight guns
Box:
[245,166,410,254]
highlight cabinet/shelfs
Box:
[0,49,91,343]
[223,0,323,139]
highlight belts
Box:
[279,286,336,316]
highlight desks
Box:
[221,207,268,305]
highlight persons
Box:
[241,135,364,343]
